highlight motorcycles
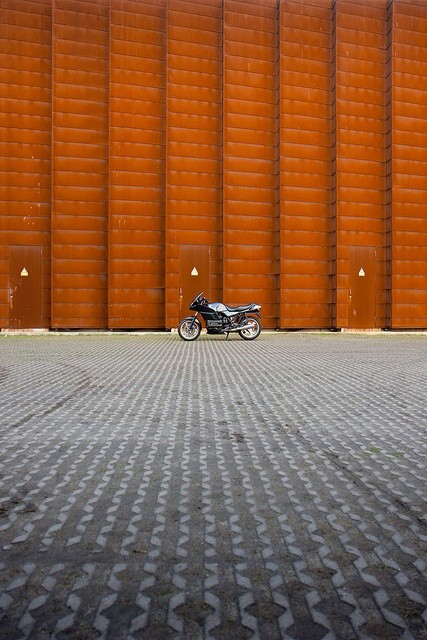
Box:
[178,292,262,341]
